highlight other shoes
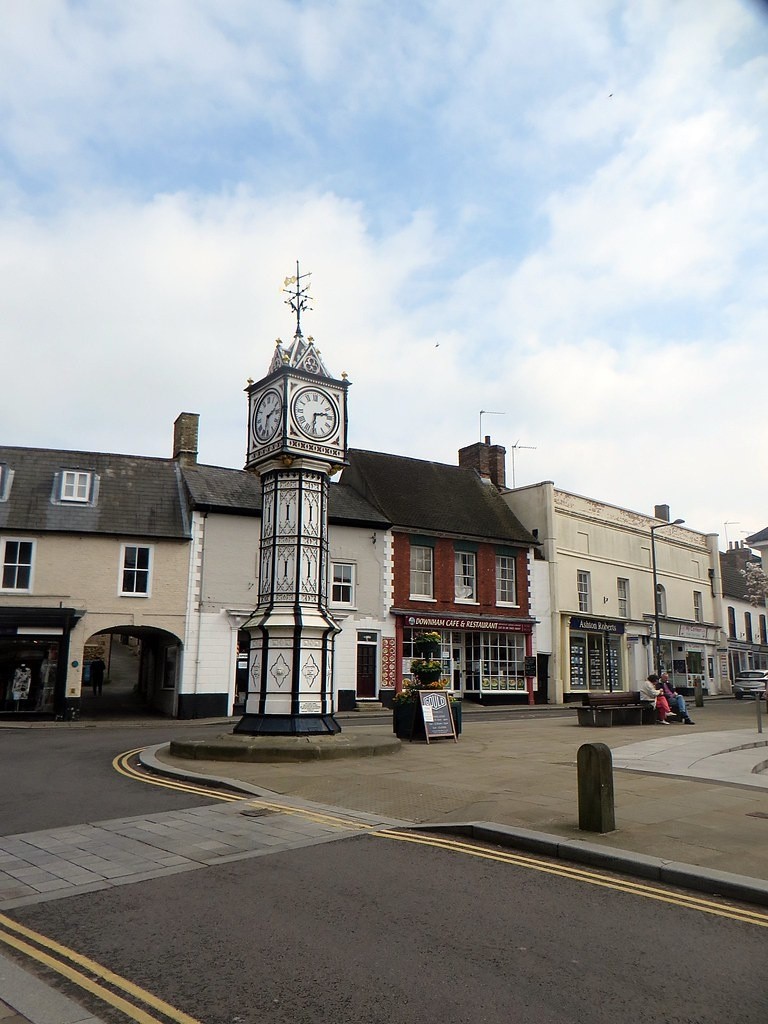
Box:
[659,719,670,724]
[684,718,695,724]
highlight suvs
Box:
[733,669,768,700]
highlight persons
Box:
[89,656,106,696]
[656,673,696,725]
[640,675,676,725]
[11,663,31,700]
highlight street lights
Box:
[649,518,685,679]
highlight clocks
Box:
[294,390,336,438]
[255,392,281,440]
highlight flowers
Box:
[410,659,444,674]
[410,632,442,643]
[391,678,458,706]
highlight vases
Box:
[414,671,441,684]
[392,702,462,743]
[415,642,438,652]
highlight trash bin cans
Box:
[83,660,93,686]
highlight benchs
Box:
[568,692,689,727]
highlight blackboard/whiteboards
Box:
[419,690,456,737]
[524,657,536,677]
